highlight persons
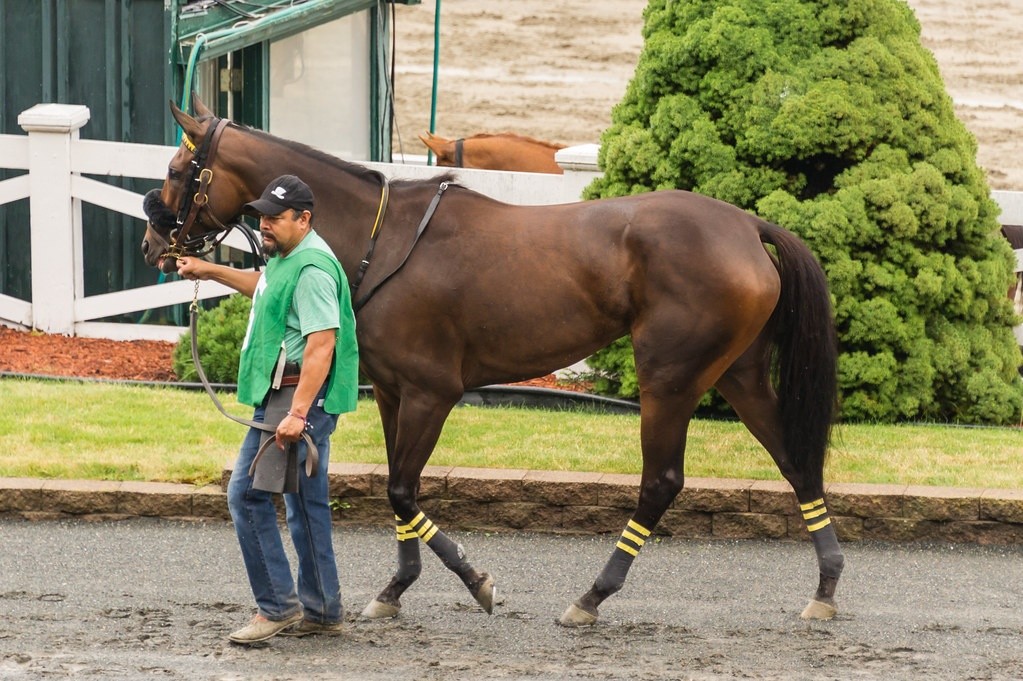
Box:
[177,175,356,639]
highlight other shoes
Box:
[278,620,343,636]
[229,612,306,643]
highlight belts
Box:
[271,374,301,388]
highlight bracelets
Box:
[286,411,314,431]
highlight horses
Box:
[141,92,846,630]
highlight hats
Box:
[243,175,314,216]
